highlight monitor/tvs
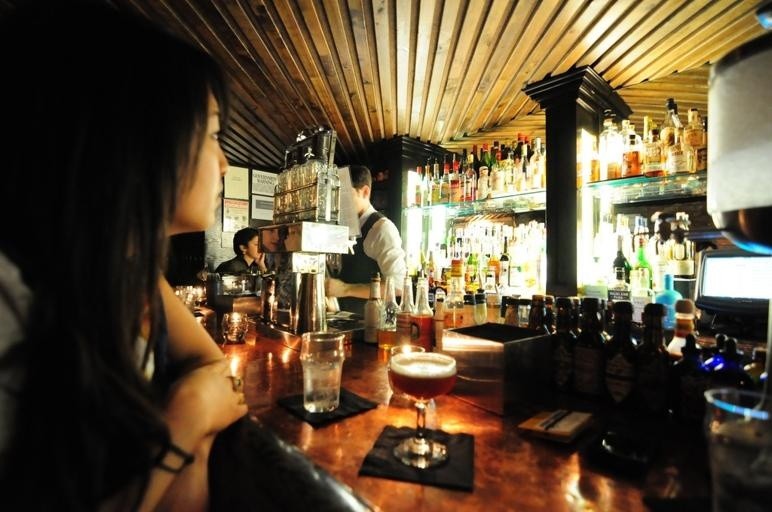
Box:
[694,251,772,342]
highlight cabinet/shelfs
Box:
[402,171,704,323]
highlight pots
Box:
[222,274,249,293]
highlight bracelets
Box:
[151,442,195,476]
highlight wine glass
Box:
[173,284,210,317]
[386,352,459,470]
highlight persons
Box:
[323,166,406,315]
[10,33,250,512]
[213,227,267,274]
[259,227,288,252]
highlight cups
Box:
[702,383,771,512]
[385,344,428,401]
[275,131,338,222]
[221,311,250,341]
[300,329,352,416]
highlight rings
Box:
[228,374,244,394]
[237,393,244,402]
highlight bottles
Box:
[357,220,545,346]
[491,292,770,419]
[583,214,699,322]
[411,98,708,212]
